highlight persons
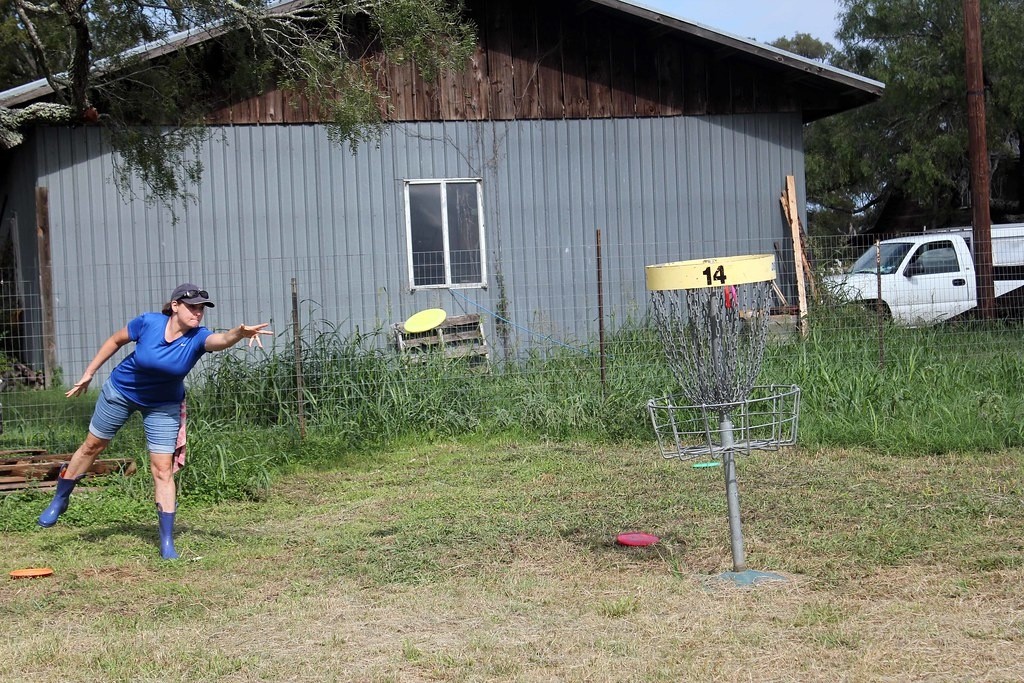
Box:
[37,283,274,560]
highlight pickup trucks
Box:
[821,222,1024,333]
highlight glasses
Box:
[177,290,208,301]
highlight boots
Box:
[155,502,179,560]
[39,463,84,527]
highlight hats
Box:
[171,283,215,308]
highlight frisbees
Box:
[617,532,660,547]
[404,308,446,334]
[692,461,722,468]
